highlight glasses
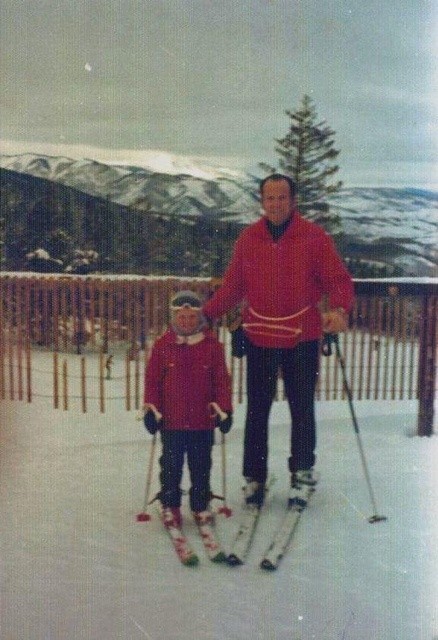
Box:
[169,295,203,311]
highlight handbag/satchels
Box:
[231,326,248,358]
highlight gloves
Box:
[215,412,232,433]
[144,410,157,434]
[321,310,347,336]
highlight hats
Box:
[170,290,207,336]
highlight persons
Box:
[142,289,232,528]
[204,173,356,510]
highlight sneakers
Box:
[193,511,211,526]
[245,478,263,507]
[288,471,316,511]
[163,508,181,529]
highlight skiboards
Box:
[227,504,302,574]
[159,505,226,568]
